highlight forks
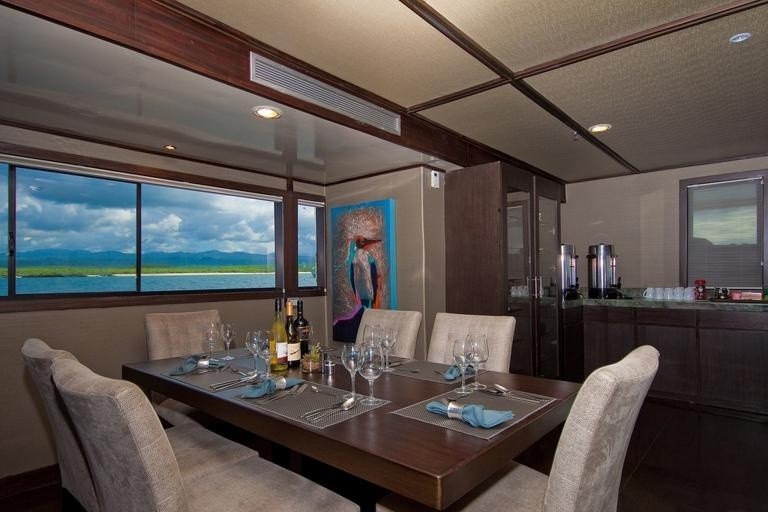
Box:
[258,383,308,404]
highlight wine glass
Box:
[340,343,364,400]
[204,322,219,361]
[357,344,385,406]
[285,300,301,367]
[363,324,383,370]
[453,340,474,393]
[220,320,238,361]
[246,332,261,375]
[270,298,289,371]
[256,329,276,378]
[294,299,309,355]
[465,331,490,390]
[380,325,397,371]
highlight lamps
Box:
[589,124,611,132]
[252,106,280,120]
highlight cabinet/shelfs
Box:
[445,161,564,376]
[636,300,698,410]
[582,299,636,378]
[697,304,767,419]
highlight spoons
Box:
[209,373,257,391]
[492,383,550,402]
[309,397,357,422]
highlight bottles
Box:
[695,279,706,300]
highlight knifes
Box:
[300,403,342,418]
[479,389,541,404]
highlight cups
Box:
[684,286,696,301]
[654,287,664,300]
[673,287,684,300]
[643,287,654,299]
[664,287,673,300]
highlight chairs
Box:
[20,338,258,511]
[354,308,422,359]
[428,312,514,372]
[51,358,358,511]
[374,348,660,512]
[144,309,225,425]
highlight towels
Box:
[425,401,514,428]
[170,355,226,376]
[242,378,306,399]
[444,362,475,380]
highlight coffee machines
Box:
[586,242,622,299]
[558,243,579,299]
